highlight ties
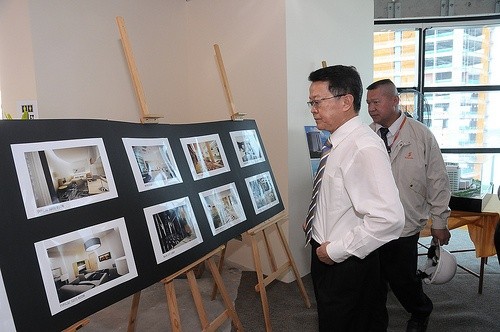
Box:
[305,140,332,246]
[380,127,389,148]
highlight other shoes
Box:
[407,301,432,332]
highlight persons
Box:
[302,65,406,332]
[366,79,452,332]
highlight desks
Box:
[417,194,499,294]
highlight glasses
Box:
[306,93,347,107]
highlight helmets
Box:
[424,237,456,285]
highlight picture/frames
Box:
[245,171,280,216]
[10,137,118,221]
[198,183,249,236]
[143,196,204,264]
[34,217,138,317]
[178,134,232,181]
[229,129,266,168]
[121,137,184,193]
